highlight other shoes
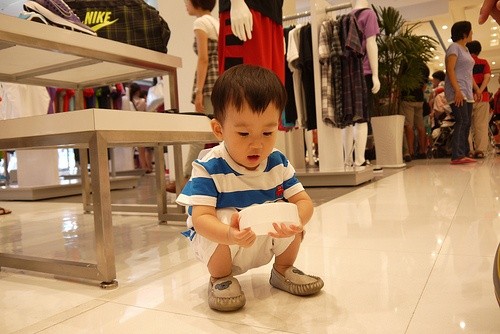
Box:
[269,263,325,295]
[207,275,246,311]
[344,160,383,174]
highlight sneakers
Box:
[475,151,484,158]
[23,0,98,36]
[461,156,477,163]
[404,153,411,162]
[467,151,475,158]
[450,158,470,164]
[416,152,427,159]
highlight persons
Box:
[0,207,12,215]
[429,70,446,159]
[166,0,221,193]
[396,36,426,161]
[128,77,164,174]
[176,64,324,312]
[217,0,285,88]
[466,40,491,160]
[444,21,475,164]
[478,0,500,308]
[345,1,383,174]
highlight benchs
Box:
[0,108,223,289]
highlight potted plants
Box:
[368,4,439,168]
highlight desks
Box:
[0,13,186,214]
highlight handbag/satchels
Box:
[64,1,171,54]
